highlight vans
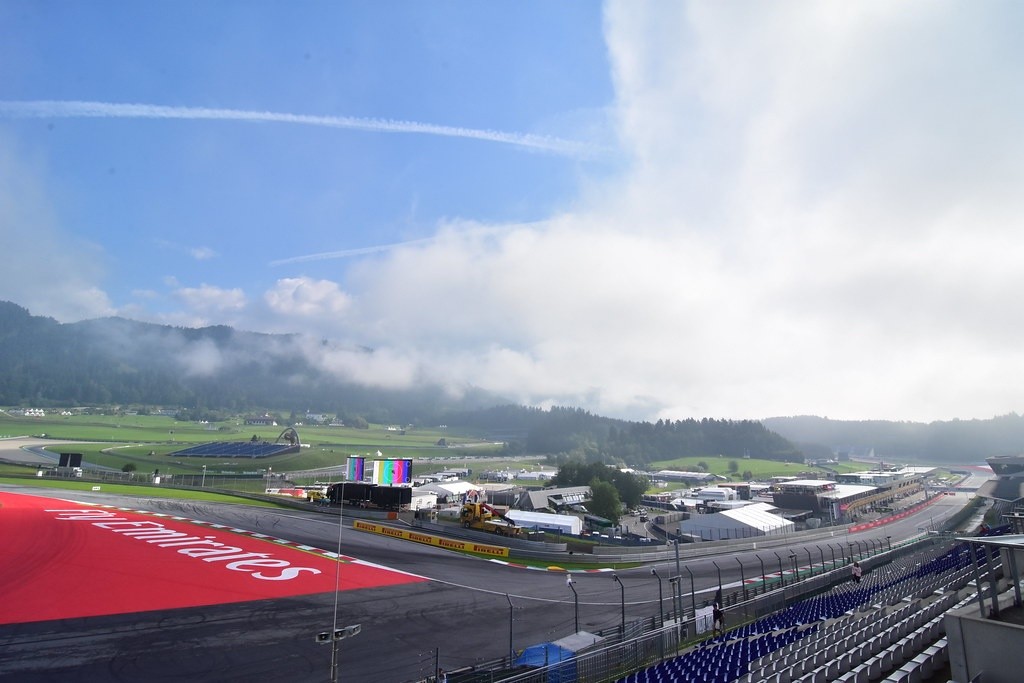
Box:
[307,491,328,501]
[631,511,640,517]
[638,509,648,515]
[640,516,649,522]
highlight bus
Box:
[265,482,338,498]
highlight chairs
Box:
[609,529,1016,683]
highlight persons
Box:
[980,523,991,532]
[712,603,724,637]
[852,562,862,583]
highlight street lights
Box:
[666,539,683,622]
[201,464,206,487]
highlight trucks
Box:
[57,467,82,478]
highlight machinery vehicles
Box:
[461,503,522,537]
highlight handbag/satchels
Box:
[715,620,720,629]
